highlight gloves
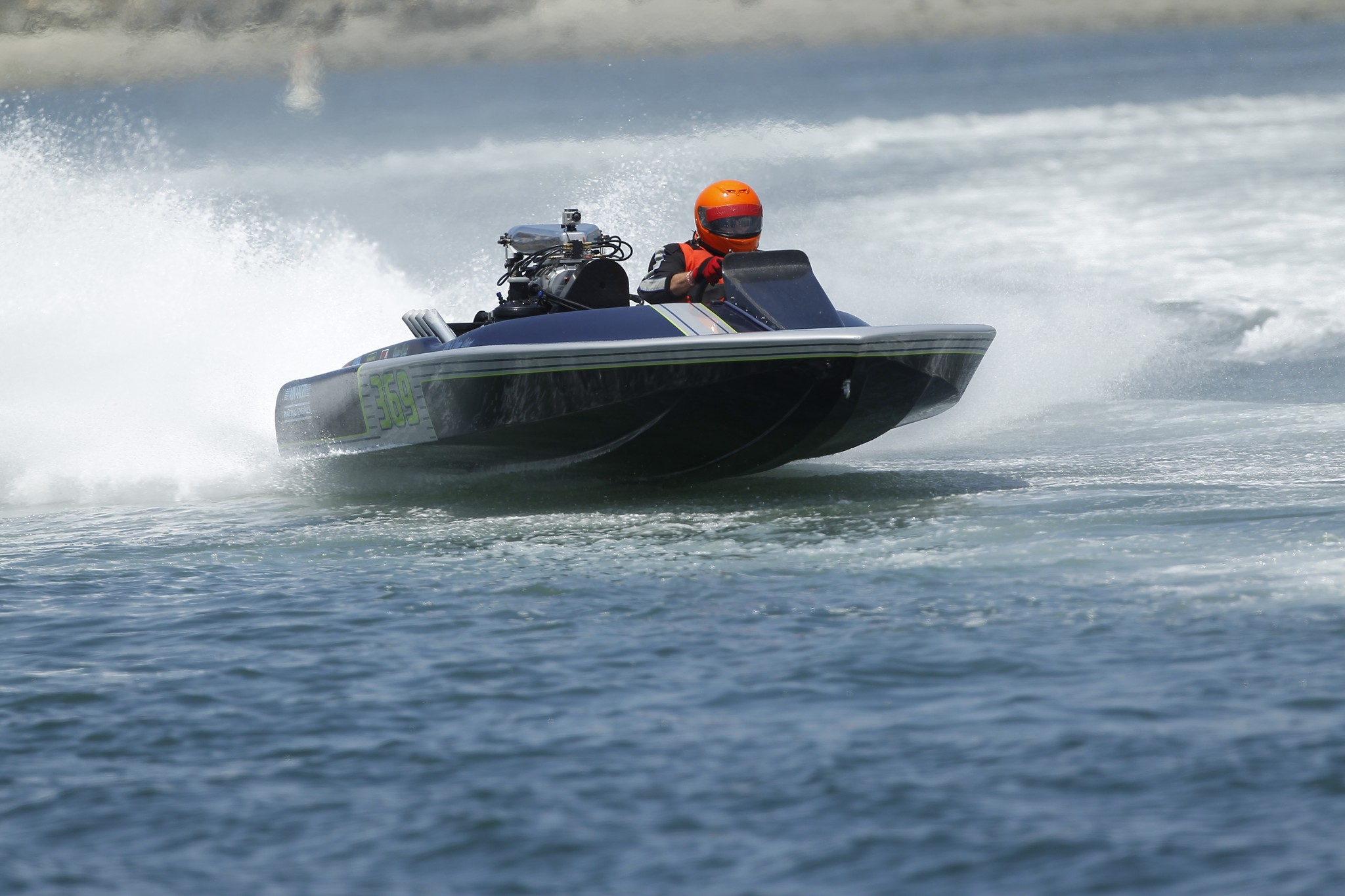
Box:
[692,256,724,286]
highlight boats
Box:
[271,206,999,488]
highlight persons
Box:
[637,179,765,305]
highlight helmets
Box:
[694,179,764,254]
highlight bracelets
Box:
[686,271,696,287]
